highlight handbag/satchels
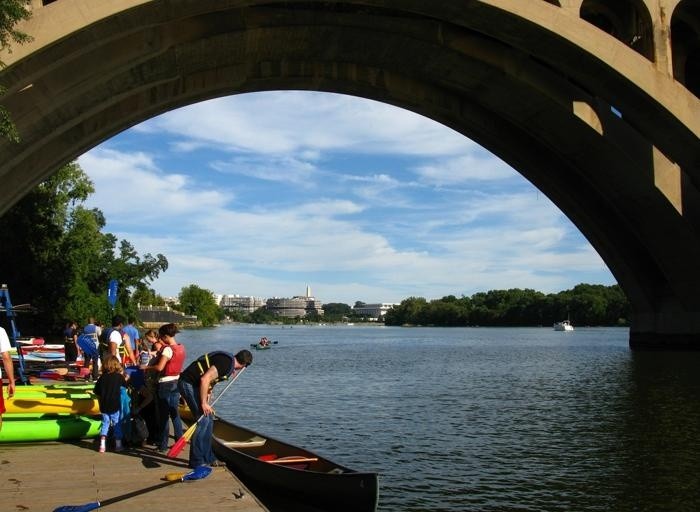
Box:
[121,414,148,448]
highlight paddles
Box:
[52,465,212,512]
[167,363,250,460]
[107,279,118,318]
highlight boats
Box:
[0,336,136,443]
[178,397,379,512]
[554,320,574,331]
[256,341,271,350]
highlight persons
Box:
[0,327,15,432]
[63,315,186,453]
[259,337,268,347]
[177,349,253,468]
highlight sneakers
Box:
[188,460,227,469]
[99,448,106,453]
[115,446,125,452]
[169,444,184,450]
[153,447,161,452]
